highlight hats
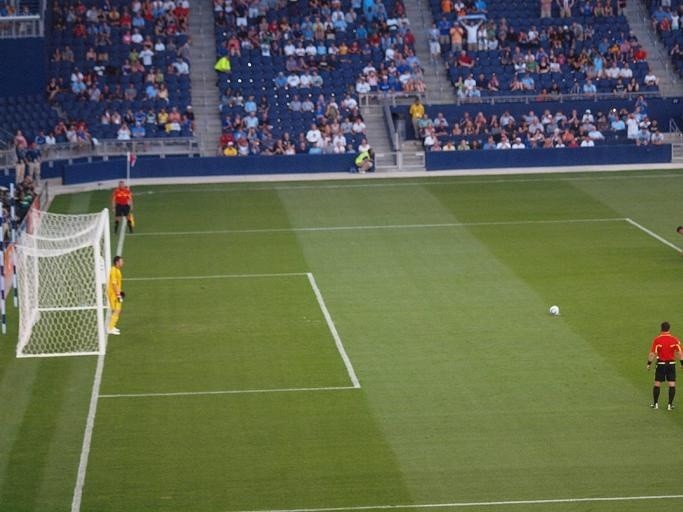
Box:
[586,110,591,112]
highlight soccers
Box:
[549,306,559,315]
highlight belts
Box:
[657,362,676,364]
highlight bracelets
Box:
[647,361,651,364]
[679,360,683,364]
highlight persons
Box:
[105,256,124,335]
[410,1,663,151]
[112,181,135,236]
[676,225,683,236]
[48,0,196,167]
[652,0,683,56]
[647,323,683,411]
[1,129,56,242]
[214,0,427,174]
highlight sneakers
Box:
[108,328,120,335]
[651,403,672,410]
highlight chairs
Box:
[428,2,661,106]
[2,1,195,152]
[212,0,425,153]
[645,2,680,74]
